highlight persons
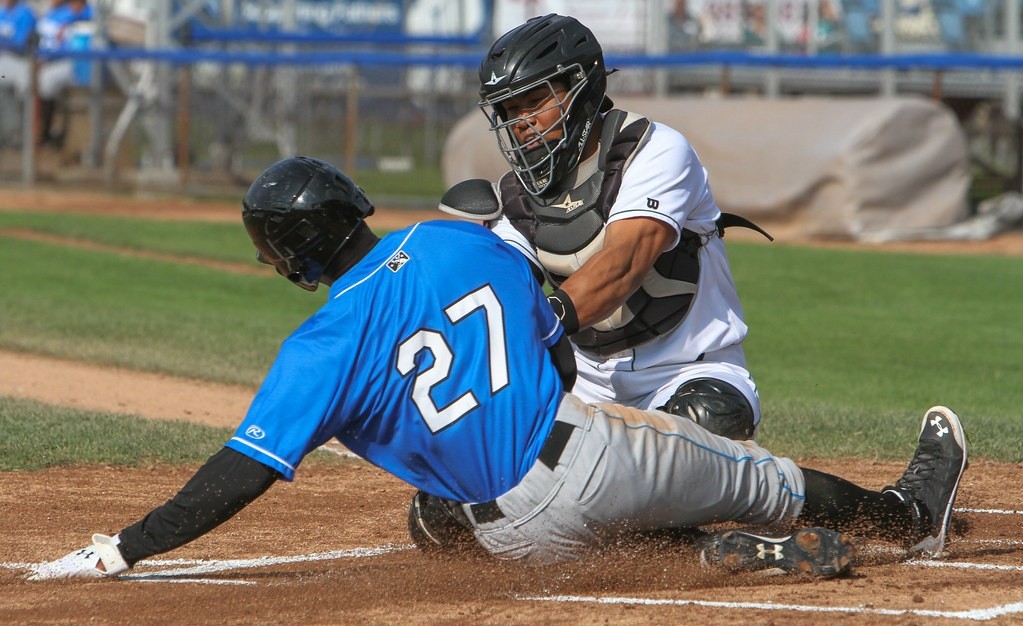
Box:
[0,0,95,138]
[407,12,761,552]
[48,155,967,581]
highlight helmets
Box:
[477,13,619,196]
[241,156,375,291]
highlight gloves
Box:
[38,534,130,581]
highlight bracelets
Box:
[545,291,581,336]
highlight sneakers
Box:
[883,406,968,558]
[699,527,855,578]
